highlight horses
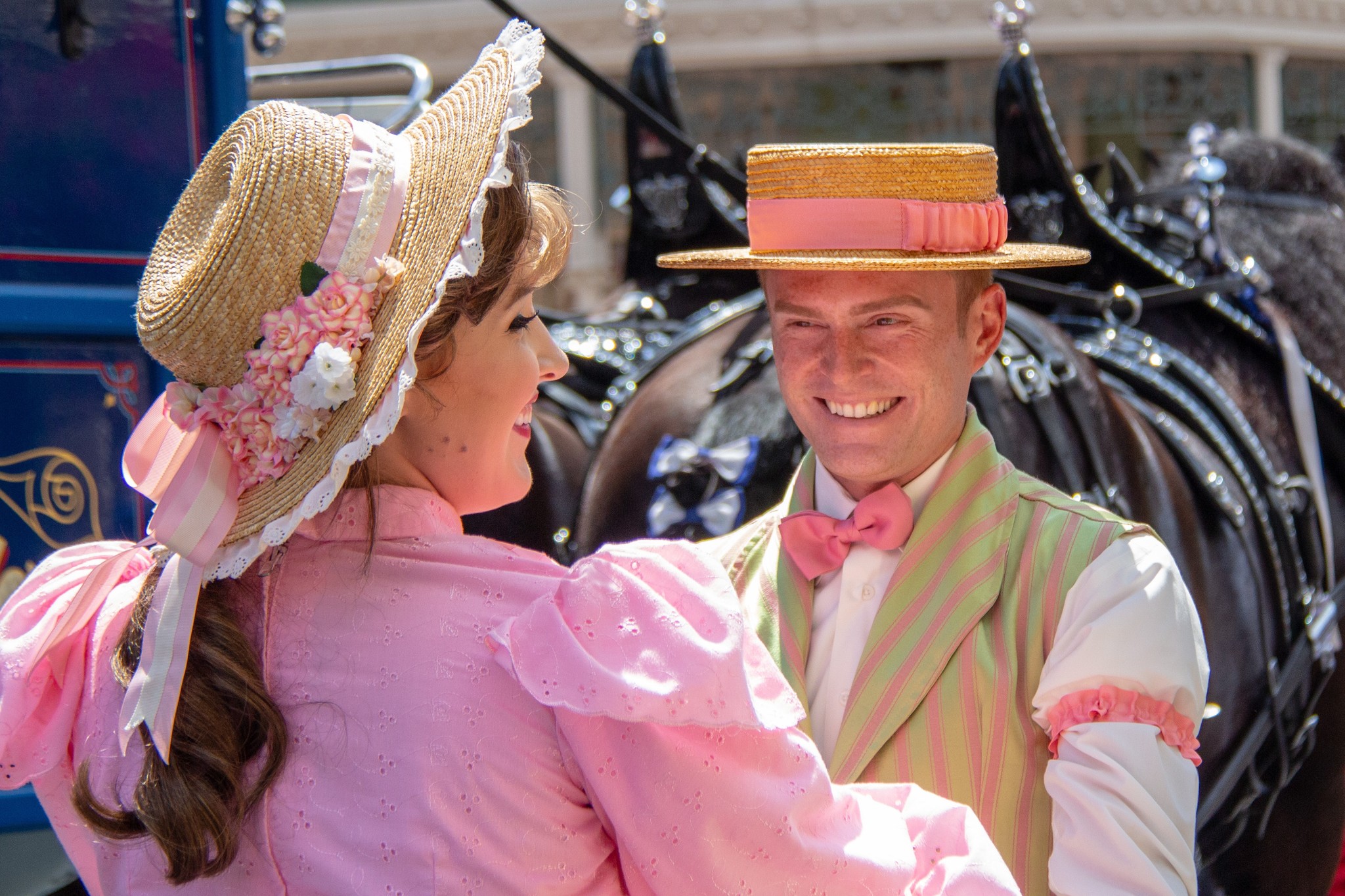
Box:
[460,163,1101,565]
[579,134,1345,896]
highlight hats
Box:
[656,142,1092,272]
[116,14,547,762]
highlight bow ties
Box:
[779,479,913,581]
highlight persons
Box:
[636,139,1210,896]
[0,16,1022,896]
